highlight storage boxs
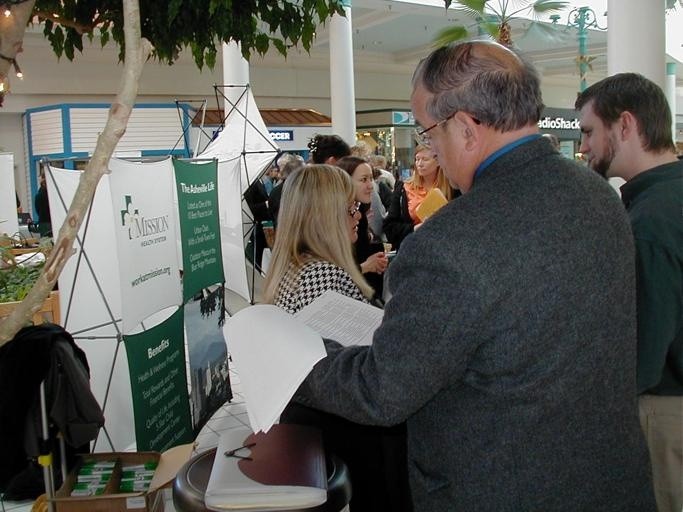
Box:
[45,440,200,512]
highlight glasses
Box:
[414,112,481,142]
[347,201,361,215]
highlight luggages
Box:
[0,324,89,504]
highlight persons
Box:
[575,73,683,450]
[373,155,396,192]
[36,181,56,238]
[336,158,388,276]
[263,164,372,315]
[307,134,353,166]
[268,160,304,232]
[293,37,658,512]
[262,167,278,196]
[403,142,451,231]
[38,173,47,189]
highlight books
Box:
[204,424,327,512]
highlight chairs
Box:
[1,290,61,326]
